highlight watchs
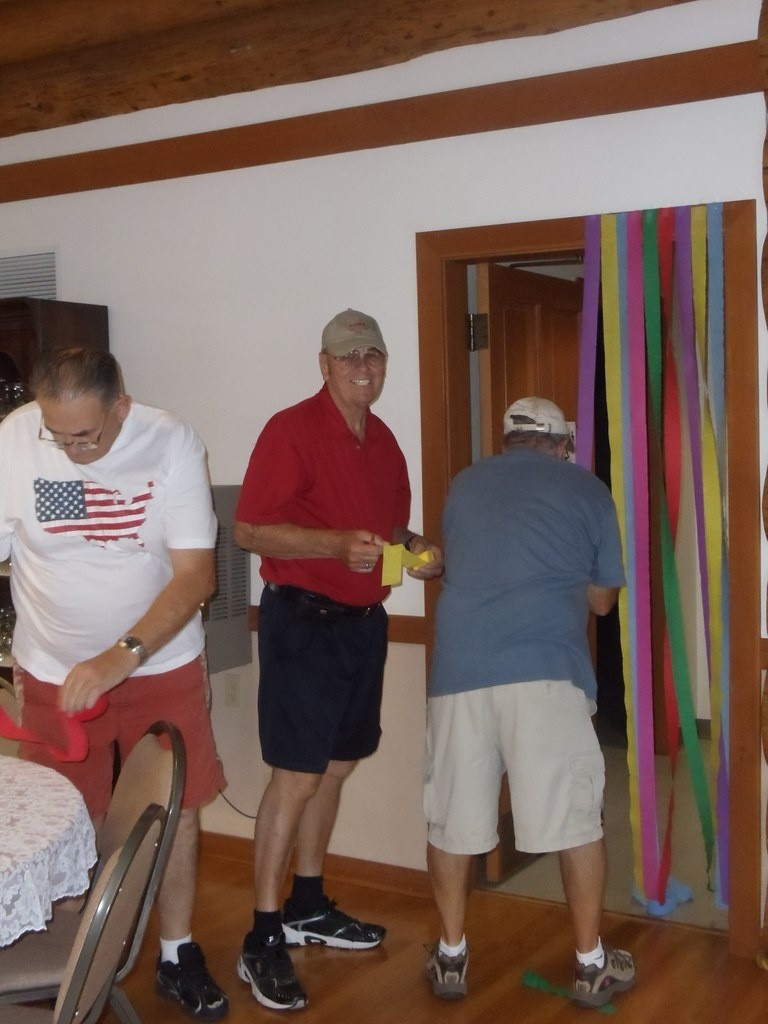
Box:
[405,533,415,551]
[116,634,149,666]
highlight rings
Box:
[364,563,371,568]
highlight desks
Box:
[0,751,96,951]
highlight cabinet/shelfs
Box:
[0,294,109,692]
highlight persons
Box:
[428,393,635,1004]
[233,311,441,1012]
[0,335,236,1024]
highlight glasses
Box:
[38,411,109,450]
[324,350,385,367]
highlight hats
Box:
[321,308,388,358]
[503,396,570,435]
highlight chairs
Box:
[0,720,185,1024]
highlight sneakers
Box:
[282,895,386,949]
[572,943,635,1006]
[157,942,229,1021]
[237,931,309,1009]
[425,940,468,999]
